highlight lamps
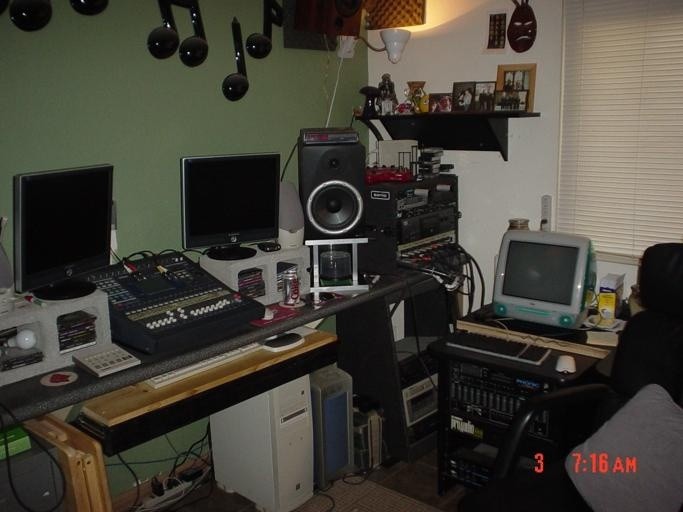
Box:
[337,29,411,64]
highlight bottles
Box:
[507,218,529,230]
[403,81,426,108]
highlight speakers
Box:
[357,231,393,276]
[367,183,393,226]
[297,137,366,238]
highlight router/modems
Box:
[257,242,281,252]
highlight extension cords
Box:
[141,480,193,509]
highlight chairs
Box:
[458,244,682,512]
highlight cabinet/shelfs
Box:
[199,241,310,306]
[0,288,113,387]
[427,302,631,496]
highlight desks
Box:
[0,251,471,512]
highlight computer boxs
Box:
[309,364,354,492]
[209,374,314,512]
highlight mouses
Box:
[261,307,274,320]
[555,353,577,374]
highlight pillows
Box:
[564,383,683,512]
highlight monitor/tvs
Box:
[492,230,597,329]
[13,164,113,301]
[180,152,280,260]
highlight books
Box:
[585,329,619,348]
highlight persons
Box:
[457,89,472,109]
[478,90,485,110]
[486,91,492,110]
[500,79,528,110]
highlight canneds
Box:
[284,268,299,303]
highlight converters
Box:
[152,481,164,496]
[183,467,203,482]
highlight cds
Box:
[40,371,78,386]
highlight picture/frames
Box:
[474,63,537,112]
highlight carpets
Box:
[290,471,446,512]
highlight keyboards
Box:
[145,342,264,390]
[445,333,552,367]
